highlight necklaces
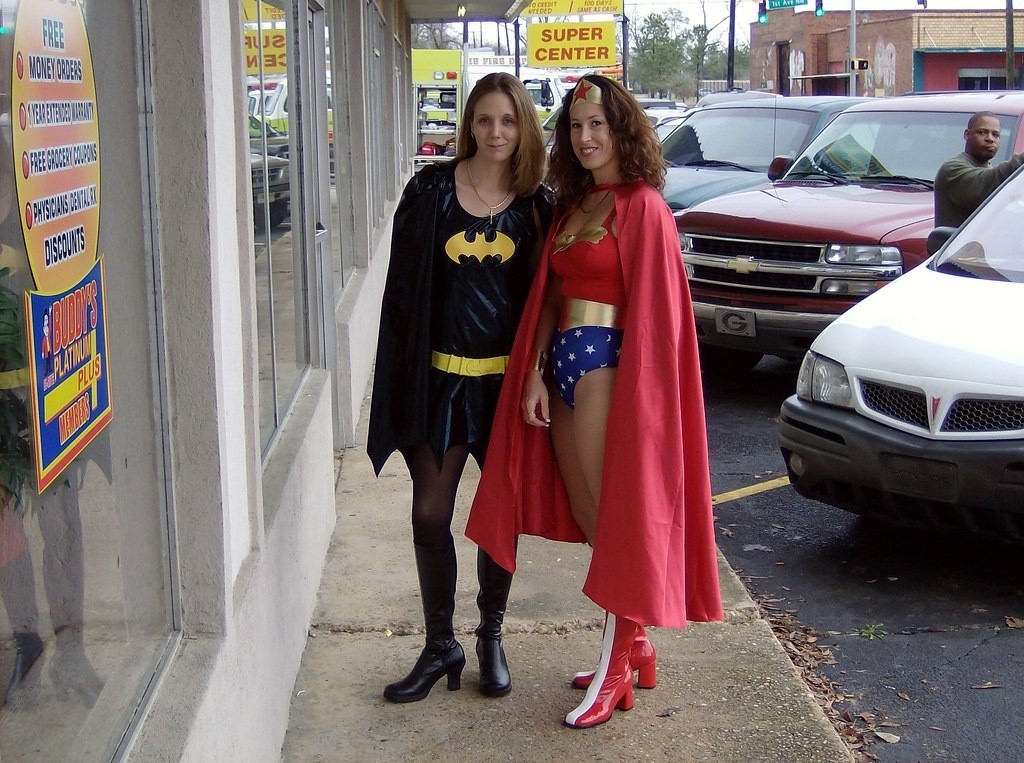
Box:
[466,159,514,225]
[580,190,612,224]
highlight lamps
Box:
[457,3,466,18]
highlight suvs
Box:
[667,85,1024,382]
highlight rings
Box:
[528,411,534,414]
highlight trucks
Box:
[409,40,581,174]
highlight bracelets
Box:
[528,350,548,376]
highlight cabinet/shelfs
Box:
[413,82,462,166]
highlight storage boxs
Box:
[423,91,457,109]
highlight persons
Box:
[648,90,661,99]
[934,111,1024,227]
[367,72,561,703]
[0,35,113,711]
[464,74,723,729]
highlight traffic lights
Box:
[857,60,869,71]
[815,0,825,17]
[850,58,859,72]
[758,0,767,24]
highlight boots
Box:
[573,623,657,688]
[475,534,512,696]
[563,611,638,729]
[383,535,467,703]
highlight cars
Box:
[631,88,878,217]
[248,112,292,160]
[246,74,335,139]
[776,160,1024,520]
[251,152,289,233]
